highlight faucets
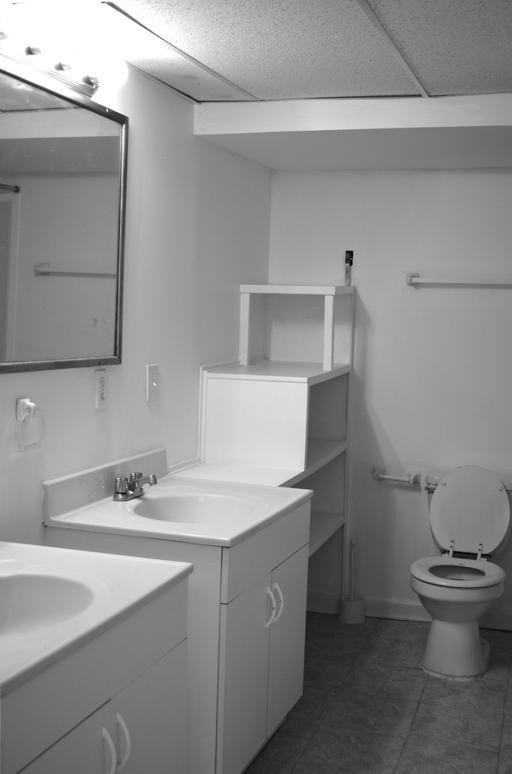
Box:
[128,473,157,498]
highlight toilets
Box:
[409,464,512,680]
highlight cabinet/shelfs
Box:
[201,286,356,617]
[0,576,190,774]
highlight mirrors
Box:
[1,53,129,375]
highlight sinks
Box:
[134,493,260,524]
[1,573,94,638]
